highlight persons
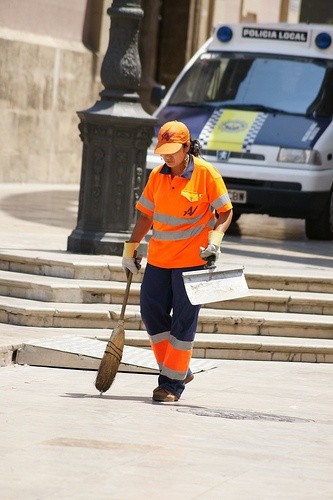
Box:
[122,122,234,402]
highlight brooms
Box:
[94,254,143,392]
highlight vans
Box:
[144,21,333,240]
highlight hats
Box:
[154,120,190,154]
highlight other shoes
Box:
[183,374,194,383]
[152,386,178,402]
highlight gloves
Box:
[199,230,225,269]
[122,240,139,278]
